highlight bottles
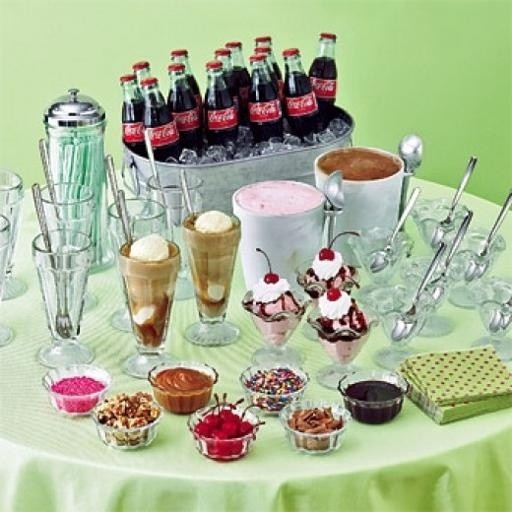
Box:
[44,88,114,273]
[121,32,343,162]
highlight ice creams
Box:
[314,276,368,364]
[243,248,302,346]
[297,232,360,304]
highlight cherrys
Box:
[194,392,266,457]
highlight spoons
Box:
[37,137,76,273]
[369,155,512,341]
[397,132,423,220]
[28,183,71,340]
[323,170,344,259]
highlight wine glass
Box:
[34,229,96,367]
[0,167,30,302]
[303,301,378,390]
[119,239,179,380]
[0,213,20,348]
[242,285,310,371]
[144,173,210,302]
[106,198,172,333]
[296,255,362,343]
[35,181,100,316]
[181,208,243,349]
[349,200,512,367]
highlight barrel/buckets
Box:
[121,105,358,232]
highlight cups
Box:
[90,397,166,451]
[234,179,328,297]
[148,360,222,414]
[338,366,410,424]
[43,363,113,419]
[278,401,353,457]
[187,405,260,463]
[312,149,405,260]
[240,366,308,417]
